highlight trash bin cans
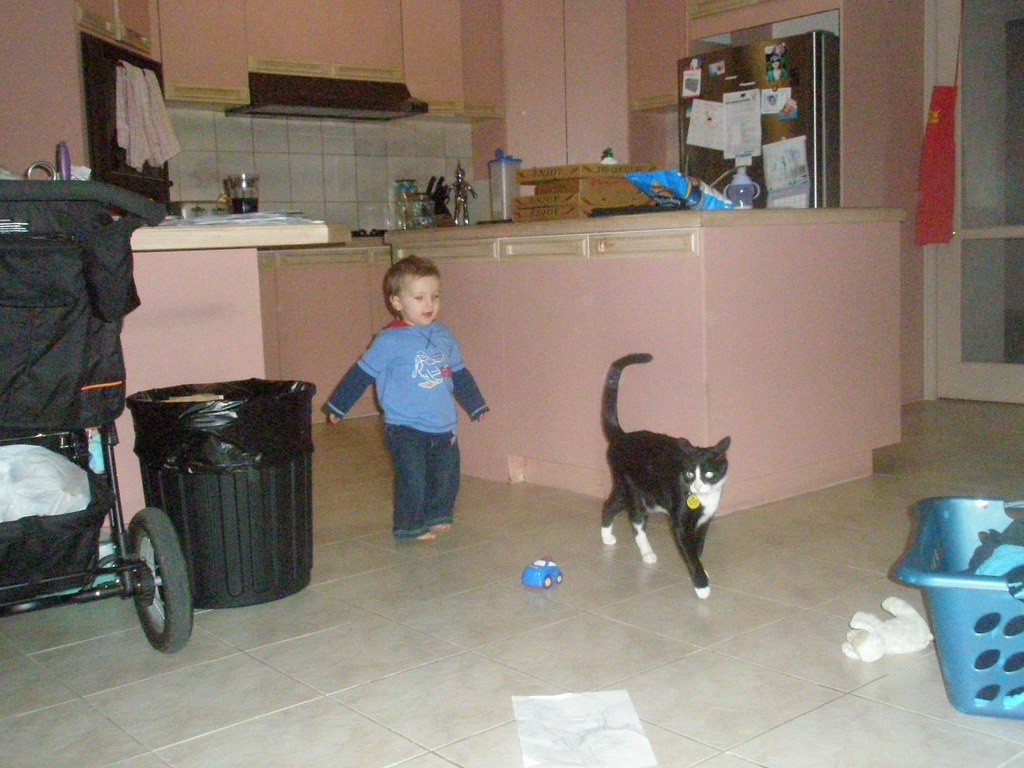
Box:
[126,377,317,610]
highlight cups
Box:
[221,173,259,214]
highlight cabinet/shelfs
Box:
[0,0,161,179]
[381,208,907,519]
[103,225,393,429]
[157,0,502,122]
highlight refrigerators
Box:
[675,30,839,209]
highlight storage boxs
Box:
[511,163,659,223]
[895,495,1024,721]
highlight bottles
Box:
[600,147,618,164]
[488,150,523,222]
[723,167,760,210]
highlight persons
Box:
[322,256,489,539]
[765,46,790,82]
[448,168,478,226]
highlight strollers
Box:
[0,175,195,656]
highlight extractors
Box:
[223,71,430,122]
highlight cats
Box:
[601,352,731,599]
